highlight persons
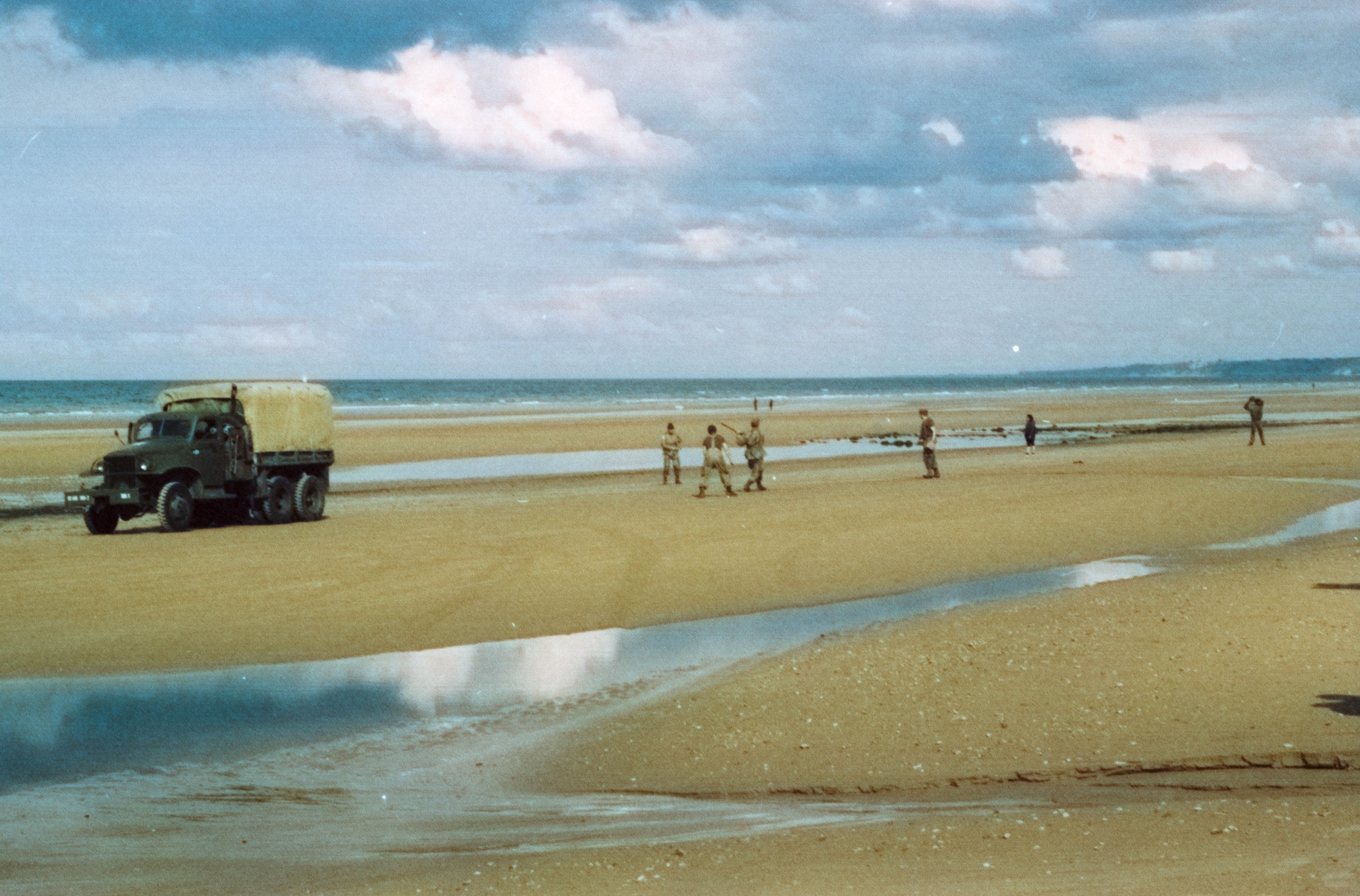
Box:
[661,422,683,485]
[1244,396,1265,446]
[737,418,767,492]
[1024,414,1036,455]
[916,409,940,479]
[692,424,738,498]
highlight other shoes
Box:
[662,476,667,485]
[923,468,940,478]
[744,480,754,492]
[724,485,737,496]
[697,488,705,498]
[757,482,766,491]
[1025,451,1035,455]
[675,476,681,484]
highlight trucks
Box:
[64,381,335,534]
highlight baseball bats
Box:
[721,423,743,435]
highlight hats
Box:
[668,421,675,429]
